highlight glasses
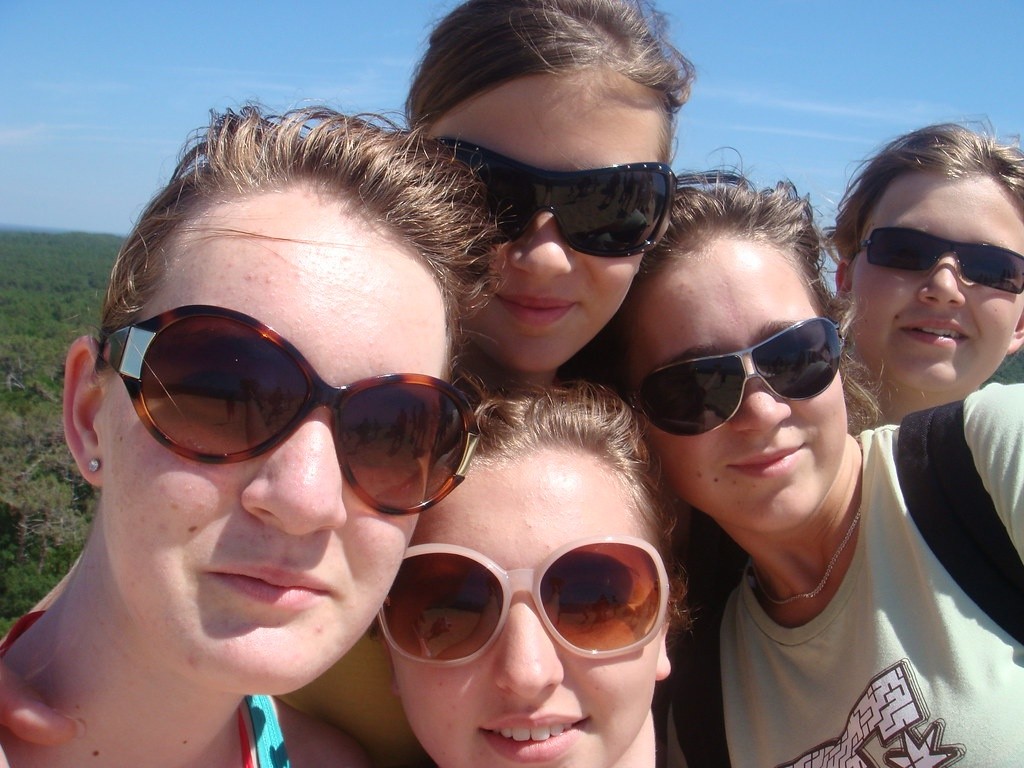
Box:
[99,304,482,516]
[859,227,1024,294]
[382,534,670,666]
[623,317,841,437]
[436,137,679,257]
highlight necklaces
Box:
[747,504,862,608]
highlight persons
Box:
[1,100,509,768]
[824,121,1024,438]
[264,0,696,768]
[569,146,1024,768]
[364,379,690,768]
